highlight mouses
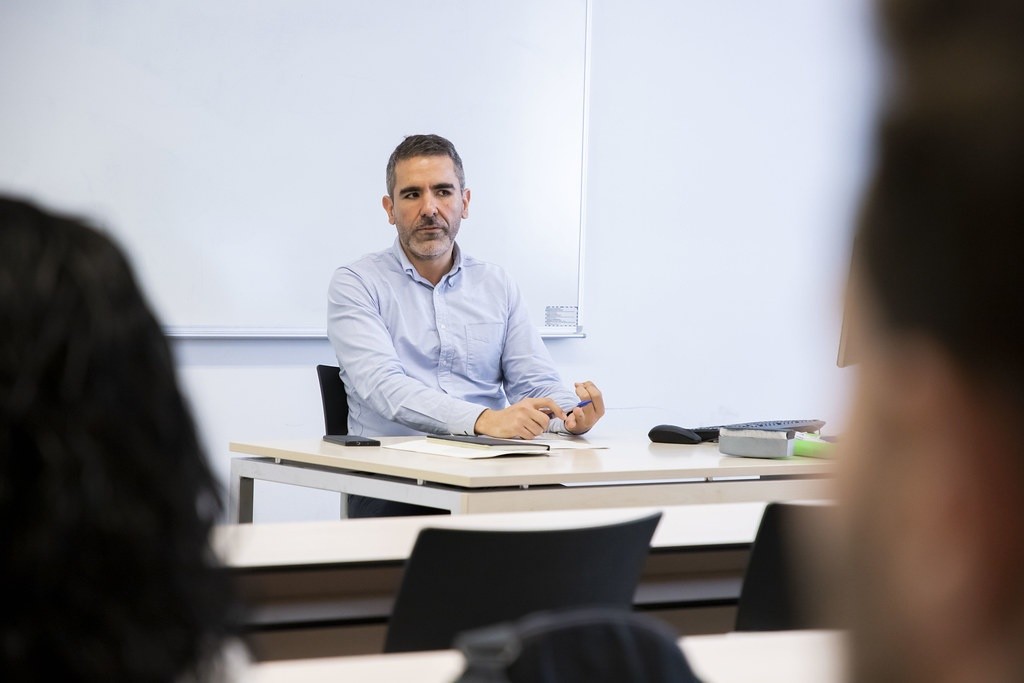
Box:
[648,425,702,444]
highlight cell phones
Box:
[322,435,381,446]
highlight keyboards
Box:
[693,419,826,440]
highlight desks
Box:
[200,498,856,683]
[230,430,851,526]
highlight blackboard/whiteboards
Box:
[0,0,587,340]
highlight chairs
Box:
[735,503,892,633]
[376,512,665,655]
[448,613,716,683]
[316,364,352,521]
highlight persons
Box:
[835,0,1024,683]
[326,133,606,519]
[0,189,256,682]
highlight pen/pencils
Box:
[563,400,592,414]
